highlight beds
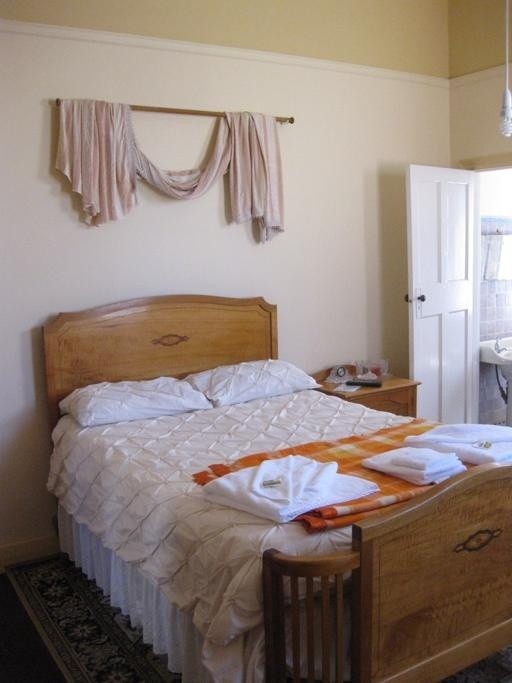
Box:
[43,295,512,683]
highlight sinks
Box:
[480,336,512,367]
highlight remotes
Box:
[346,380,382,387]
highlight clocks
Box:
[324,366,353,383]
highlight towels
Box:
[393,447,459,469]
[359,445,466,486]
[404,424,512,466]
[202,453,381,524]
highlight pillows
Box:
[61,376,213,427]
[182,359,322,408]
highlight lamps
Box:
[500,87,512,136]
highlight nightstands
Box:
[318,376,422,418]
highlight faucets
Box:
[493,337,509,353]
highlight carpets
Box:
[0,556,512,683]
